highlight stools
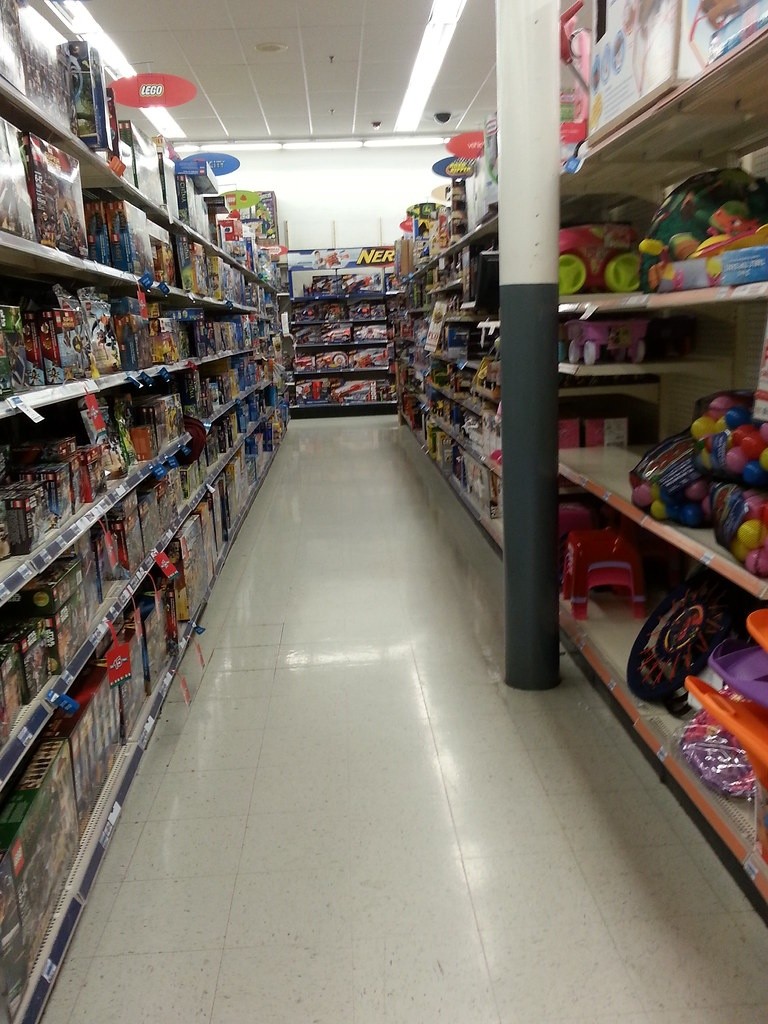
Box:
[563,525,647,621]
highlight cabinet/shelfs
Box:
[396,24,768,927]
[0,75,291,1024]
[278,291,405,419]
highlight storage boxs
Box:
[0,0,768,1024]
